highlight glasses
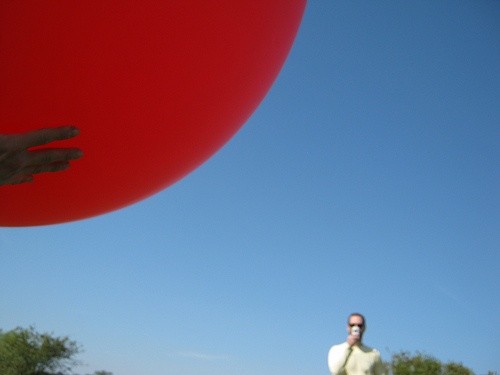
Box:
[349,323,363,328]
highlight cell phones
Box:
[352,325,360,335]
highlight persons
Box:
[0,119,81,184]
[327,310,383,375]
